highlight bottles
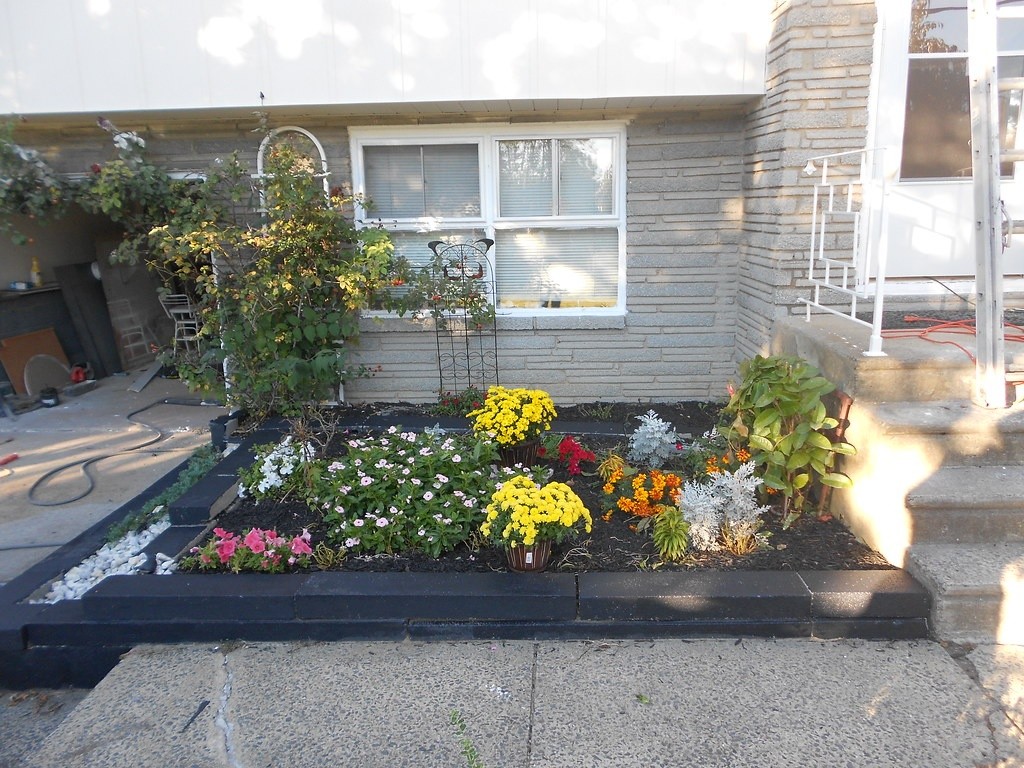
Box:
[30,257,43,287]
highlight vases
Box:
[498,439,540,469]
[503,540,552,571]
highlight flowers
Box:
[466,384,557,446]
[480,475,593,548]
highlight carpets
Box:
[845,310,1024,330]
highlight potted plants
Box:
[144,134,394,451]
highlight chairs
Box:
[107,299,148,371]
[158,295,213,357]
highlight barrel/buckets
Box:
[40,387,59,408]
